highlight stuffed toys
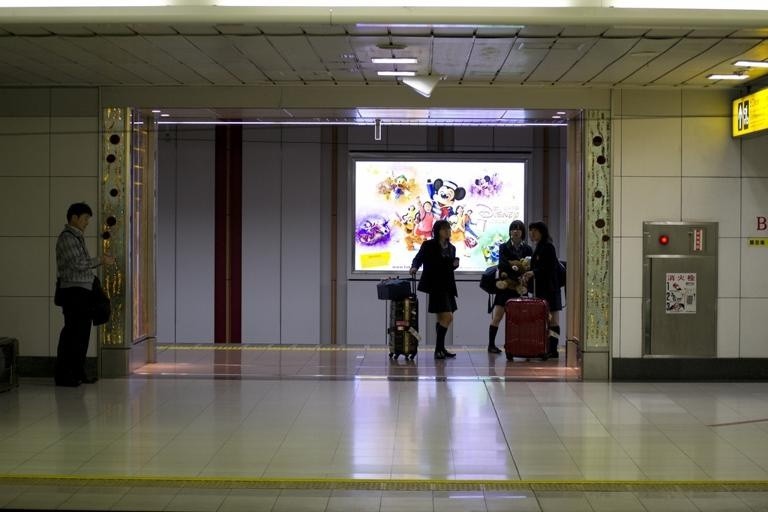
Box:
[495,256,531,295]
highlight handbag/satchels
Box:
[54,278,63,307]
[548,263,566,288]
[480,264,499,294]
[376,278,412,300]
[92,278,111,326]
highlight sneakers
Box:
[65,377,94,387]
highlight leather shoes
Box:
[489,346,502,353]
[444,351,457,357]
[550,351,560,358]
[434,351,446,359]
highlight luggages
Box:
[504,277,549,361]
[389,271,419,359]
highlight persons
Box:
[409,220,459,359]
[54,203,115,387]
[414,196,437,241]
[488,220,534,353]
[524,221,562,362]
[464,210,480,240]
[447,205,471,258]
